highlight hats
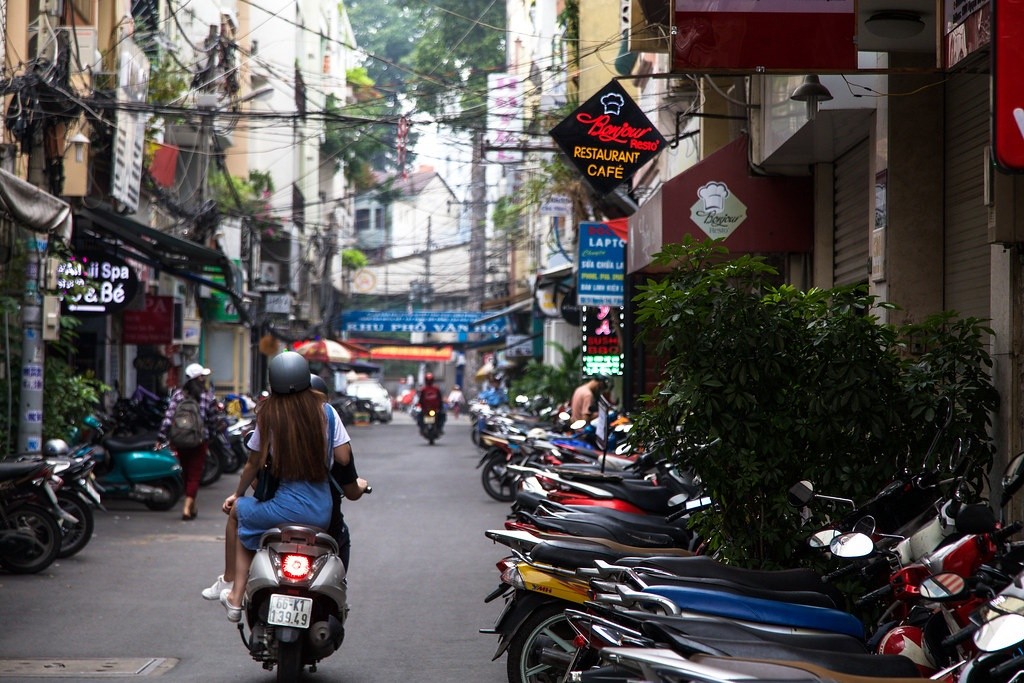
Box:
[185,363,210,380]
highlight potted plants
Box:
[246,168,275,218]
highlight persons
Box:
[156,364,219,520]
[202,351,368,623]
[398,373,508,434]
[571,374,606,431]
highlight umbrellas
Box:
[296,337,358,364]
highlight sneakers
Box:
[220,588,241,622]
[201,575,234,600]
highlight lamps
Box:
[67,113,92,163]
[791,74,834,121]
[863,13,928,38]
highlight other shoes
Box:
[183,507,198,520]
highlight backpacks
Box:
[170,387,207,449]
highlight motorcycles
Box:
[422,408,442,445]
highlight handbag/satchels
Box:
[252,461,276,501]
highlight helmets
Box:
[268,351,310,393]
[425,373,433,381]
[598,370,615,394]
[310,374,328,394]
[44,438,69,455]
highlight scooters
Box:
[470,392,1023,683]
[46,375,259,511]
[237,484,367,683]
[332,395,357,424]
[1,440,107,576]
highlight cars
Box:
[391,389,422,419]
[346,380,392,424]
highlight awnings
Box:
[471,300,533,328]
[630,130,810,276]
